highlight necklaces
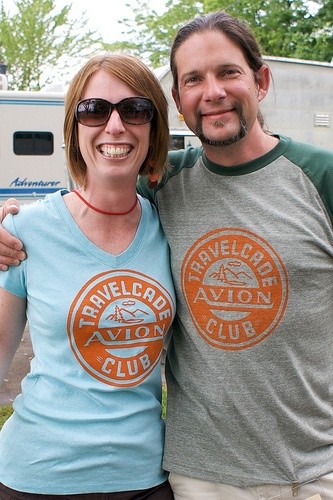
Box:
[72,189,138,215]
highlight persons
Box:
[0,11,333,500]
[0,51,178,500]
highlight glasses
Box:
[73,97,155,128]
